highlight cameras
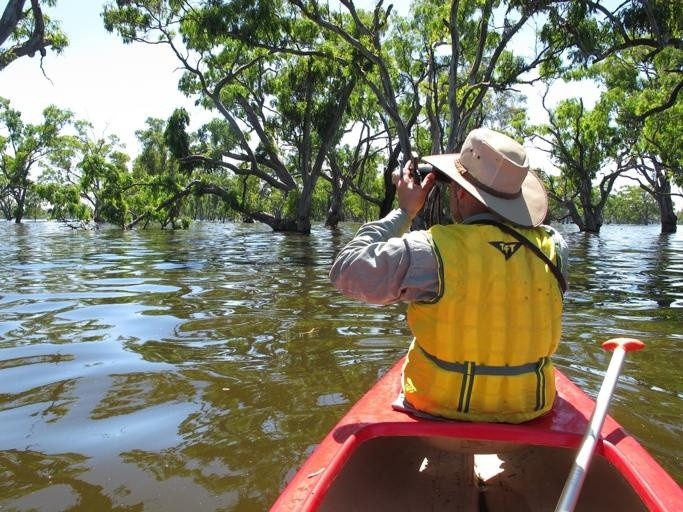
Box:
[411,150,453,185]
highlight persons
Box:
[328,127,569,426]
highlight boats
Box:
[268,355,683,511]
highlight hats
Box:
[421,129,548,229]
